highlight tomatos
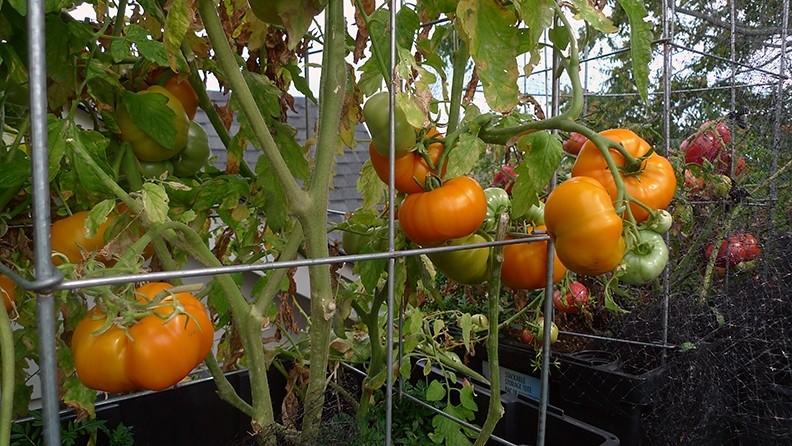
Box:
[0,69,214,391]
[343,92,759,345]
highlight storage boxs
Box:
[12,317,667,446]
